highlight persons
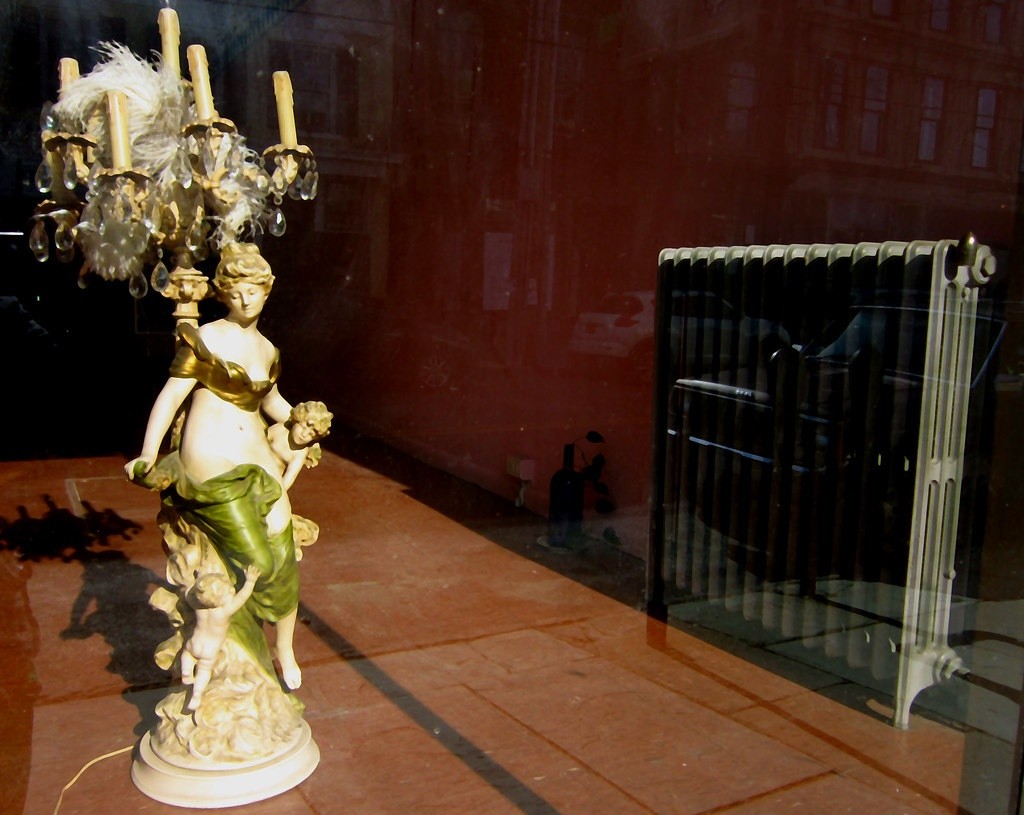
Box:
[119,254,307,694]
[176,561,263,712]
[262,400,332,492]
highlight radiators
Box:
[642,229,998,732]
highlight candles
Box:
[185,44,218,121]
[156,7,183,79]
[271,68,304,146]
[60,55,80,94]
[103,88,135,170]
